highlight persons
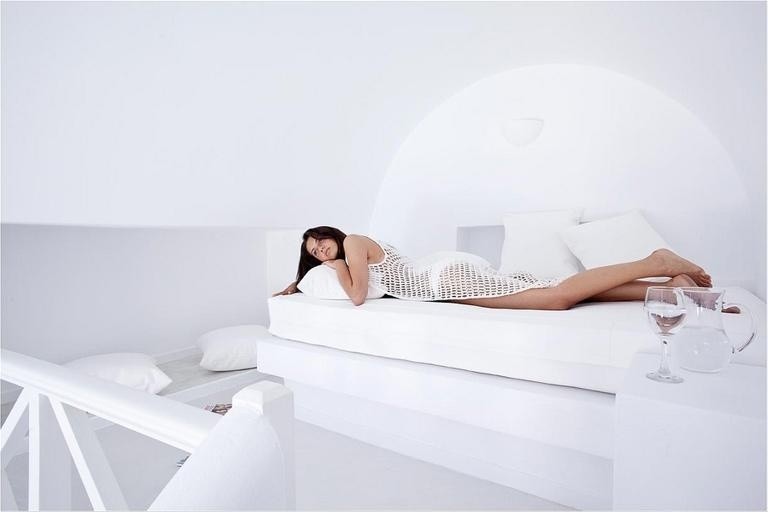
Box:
[272,226,740,313]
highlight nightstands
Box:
[607,346,765,508]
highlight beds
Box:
[257,279,766,509]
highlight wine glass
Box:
[645,286,686,384]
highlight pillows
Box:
[59,321,271,392]
[498,208,579,278]
[294,256,389,303]
[558,207,666,277]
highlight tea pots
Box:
[669,287,757,373]
